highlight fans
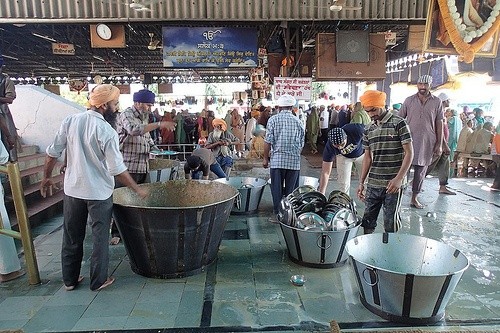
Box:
[299,0,362,13]
[104,0,158,12]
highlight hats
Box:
[328,128,347,145]
[417,75,433,84]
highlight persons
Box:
[0,139,26,282]
[110,88,178,245]
[0,66,23,161]
[409,91,457,194]
[397,74,443,208]
[357,89,414,234]
[149,92,499,189]
[262,94,305,222]
[318,122,371,211]
[40,83,147,290]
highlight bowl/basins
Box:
[144,158,180,182]
[346,232,470,325]
[111,179,242,279]
[276,214,363,269]
[213,177,268,215]
[290,275,307,287]
[267,174,319,198]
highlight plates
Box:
[279,184,359,232]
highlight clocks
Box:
[96,23,112,41]
